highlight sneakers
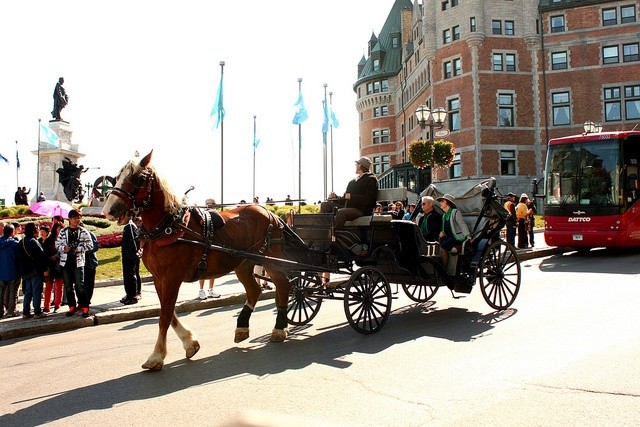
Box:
[23,314,34,318]
[81,307,90,317]
[199,289,207,300]
[34,312,48,318]
[120,297,137,305]
[66,306,78,316]
[53,308,60,313]
[42,308,50,312]
[207,289,220,298]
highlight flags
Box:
[252,120,260,149]
[291,82,308,125]
[322,96,339,133]
[16,148,20,171]
[210,66,225,128]
[0,154,11,165]
[39,123,60,148]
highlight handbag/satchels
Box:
[524,215,531,231]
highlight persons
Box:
[515,197,533,248]
[320,156,379,230]
[134,216,144,301]
[75,219,99,309]
[0,224,20,316]
[51,215,69,306]
[120,214,138,304]
[394,201,404,220]
[3,223,24,313]
[50,77,69,122]
[284,195,293,205]
[373,202,382,215]
[54,210,94,318]
[418,196,444,255]
[403,203,416,220]
[436,194,474,252]
[14,223,50,320]
[518,193,537,247]
[37,191,47,201]
[42,222,64,312]
[73,164,89,200]
[21,186,31,206]
[381,203,398,219]
[14,187,22,205]
[503,192,520,250]
[37,225,48,299]
[253,196,259,204]
[198,199,222,300]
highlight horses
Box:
[100,150,290,370]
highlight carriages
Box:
[102,150,520,370]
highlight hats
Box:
[355,156,372,170]
[507,192,516,199]
[436,193,457,209]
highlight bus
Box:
[530,131,640,252]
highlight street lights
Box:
[584,120,603,133]
[85,182,92,199]
[415,105,447,168]
